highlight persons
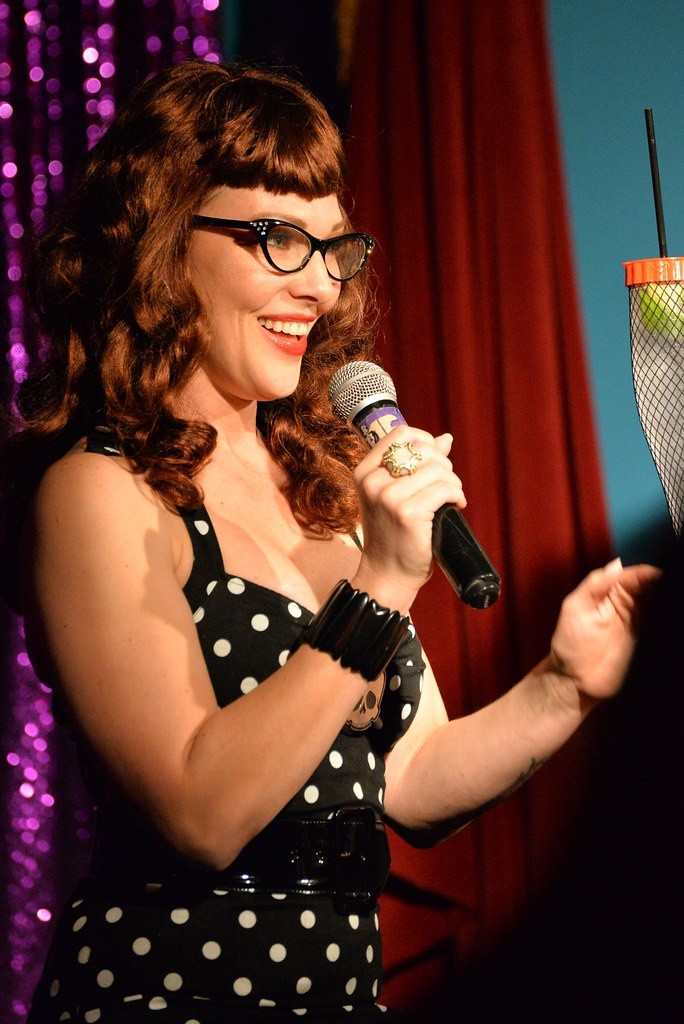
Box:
[8,63,665,1022]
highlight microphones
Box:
[327,360,501,609]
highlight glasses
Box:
[188,213,375,282]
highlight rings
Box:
[381,441,422,478]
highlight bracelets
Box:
[298,580,411,681]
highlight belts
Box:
[91,810,389,919]
